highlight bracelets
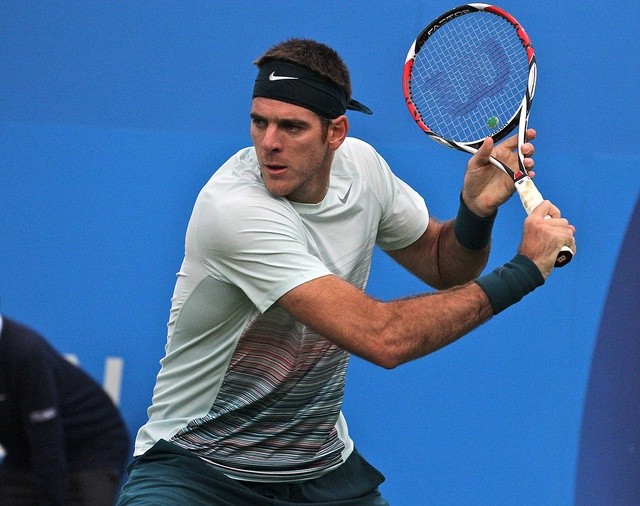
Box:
[453,191,499,253]
[476,253,545,312]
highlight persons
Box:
[117,38,576,504]
